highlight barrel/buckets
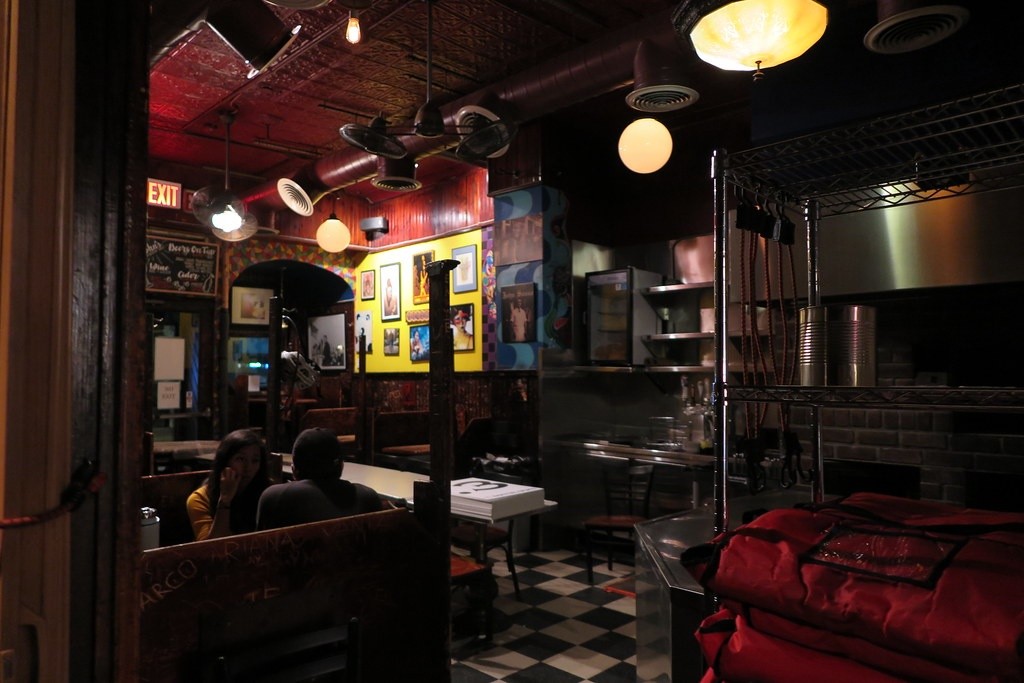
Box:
[799,305,878,387]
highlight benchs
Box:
[139,404,505,682]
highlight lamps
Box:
[412,0,445,138]
[618,117,673,174]
[214,112,241,229]
[673,0,829,82]
[316,193,350,253]
[347,0,361,44]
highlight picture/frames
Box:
[302,244,536,369]
[230,286,273,325]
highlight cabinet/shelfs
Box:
[580,269,773,375]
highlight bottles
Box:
[140,505,160,549]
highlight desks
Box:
[280,457,560,599]
[382,444,431,459]
[151,441,222,470]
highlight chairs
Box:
[442,432,535,596]
[584,453,657,582]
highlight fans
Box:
[338,113,521,162]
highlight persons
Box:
[363,276,372,296]
[508,289,531,340]
[384,279,398,316]
[256,429,384,529]
[411,331,423,356]
[452,306,473,350]
[414,255,429,295]
[186,428,282,540]
[356,314,372,351]
[388,331,394,350]
[312,335,344,366]
